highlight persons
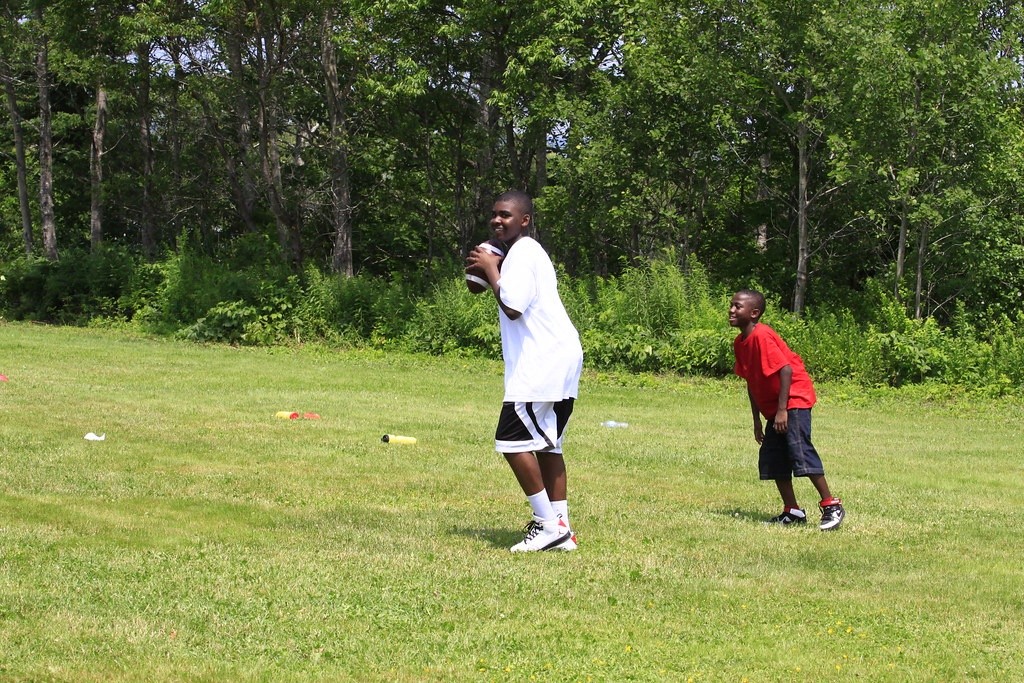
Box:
[728,289,845,531]
[465,190,583,552]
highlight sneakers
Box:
[818,497,845,530]
[766,503,806,525]
[509,511,572,552]
[545,532,578,551]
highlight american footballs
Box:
[465,237,508,294]
